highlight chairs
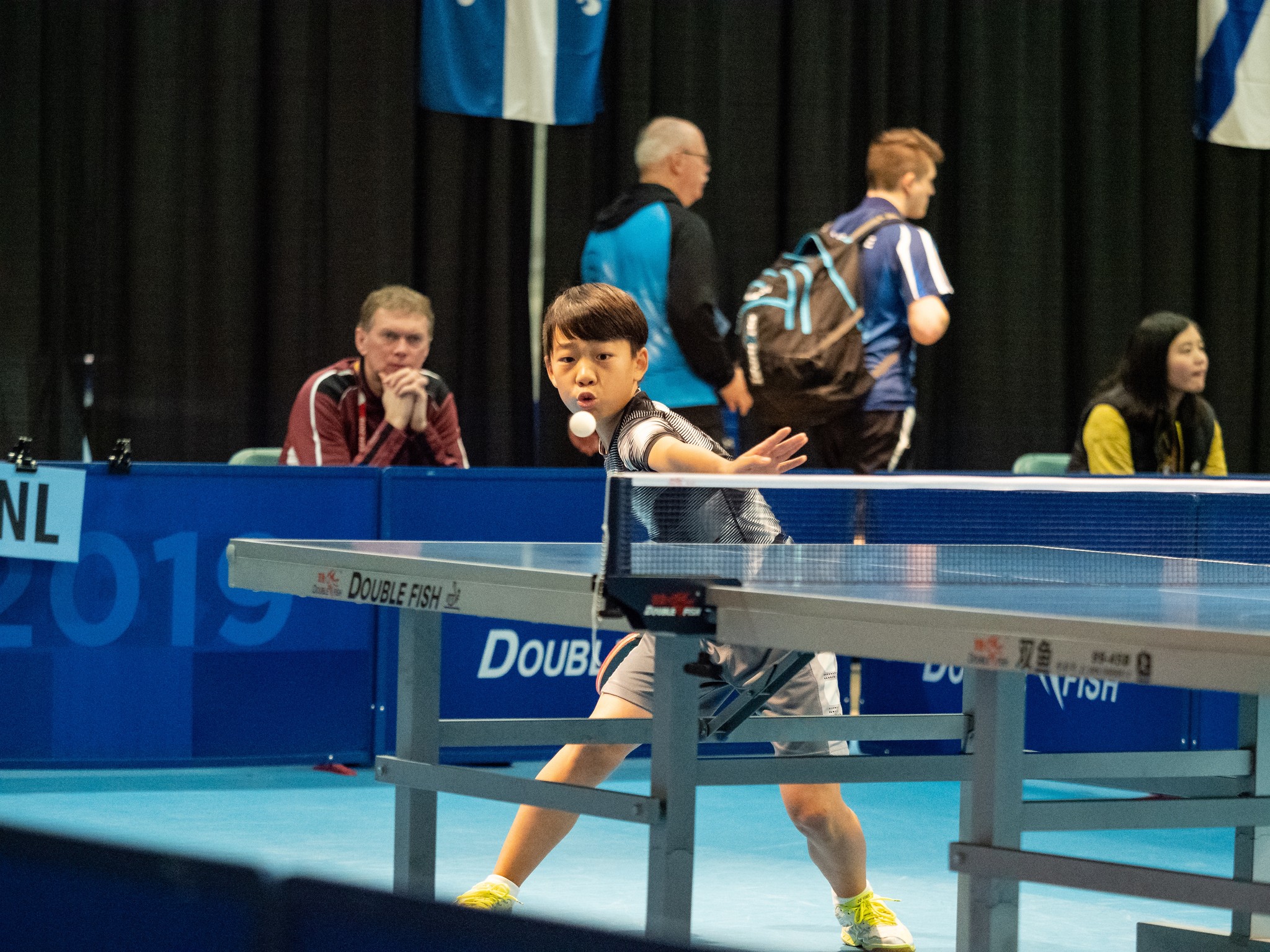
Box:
[226,445,283,466]
[1012,451,1073,475]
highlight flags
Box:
[1195,0,1270,151]
[420,0,610,125]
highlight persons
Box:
[278,286,470,469]
[579,116,753,454]
[454,281,917,952]
[1066,312,1228,476]
[793,126,952,474]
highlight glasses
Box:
[685,151,715,166]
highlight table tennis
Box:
[569,411,597,437]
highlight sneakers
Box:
[834,889,914,952]
[453,882,523,916]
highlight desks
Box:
[223,466,1270,952]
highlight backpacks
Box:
[737,212,910,423]
